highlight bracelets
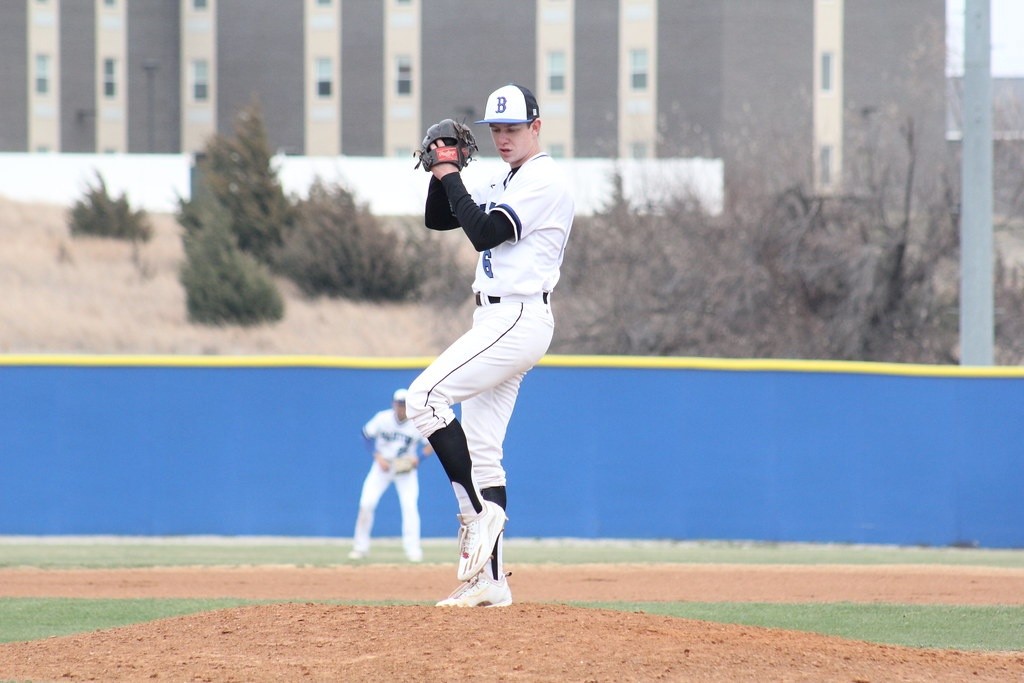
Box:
[417,450,426,462]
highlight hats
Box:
[473,83,540,124]
[393,388,407,403]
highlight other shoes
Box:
[347,549,366,560]
[408,551,422,562]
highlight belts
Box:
[474,291,550,306]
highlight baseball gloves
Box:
[418,117,479,172]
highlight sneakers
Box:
[434,571,513,609]
[456,496,507,582]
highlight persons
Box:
[349,389,437,564]
[403,84,575,609]
